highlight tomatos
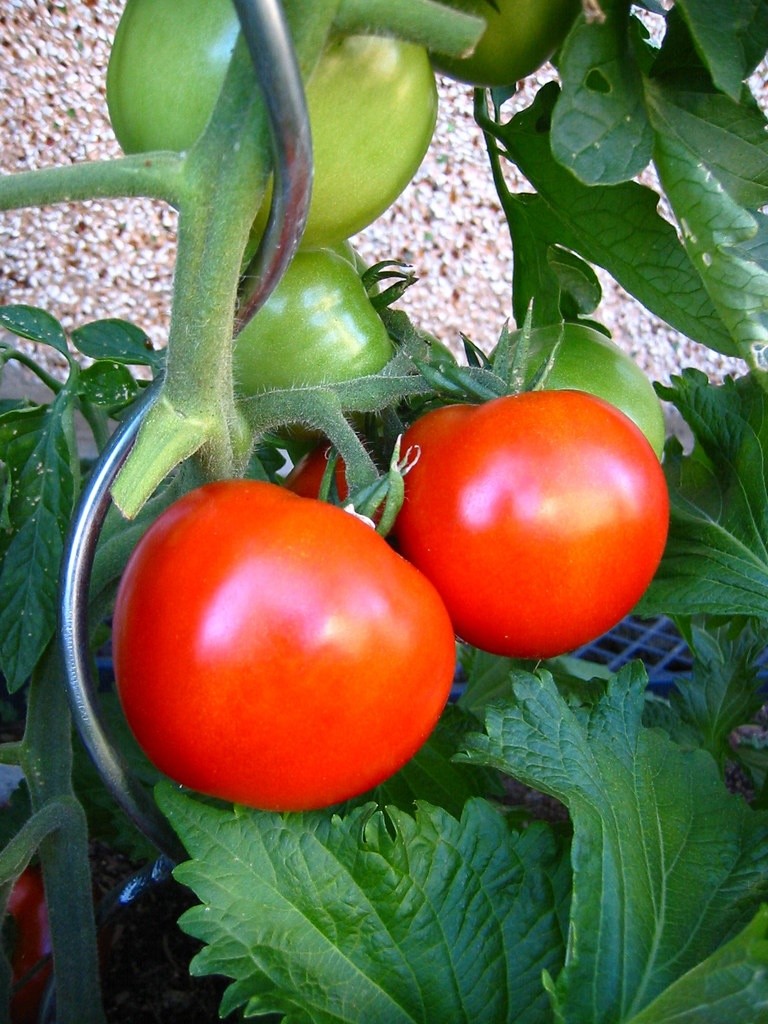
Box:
[0,867,56,1024]
[101,0,668,811]
